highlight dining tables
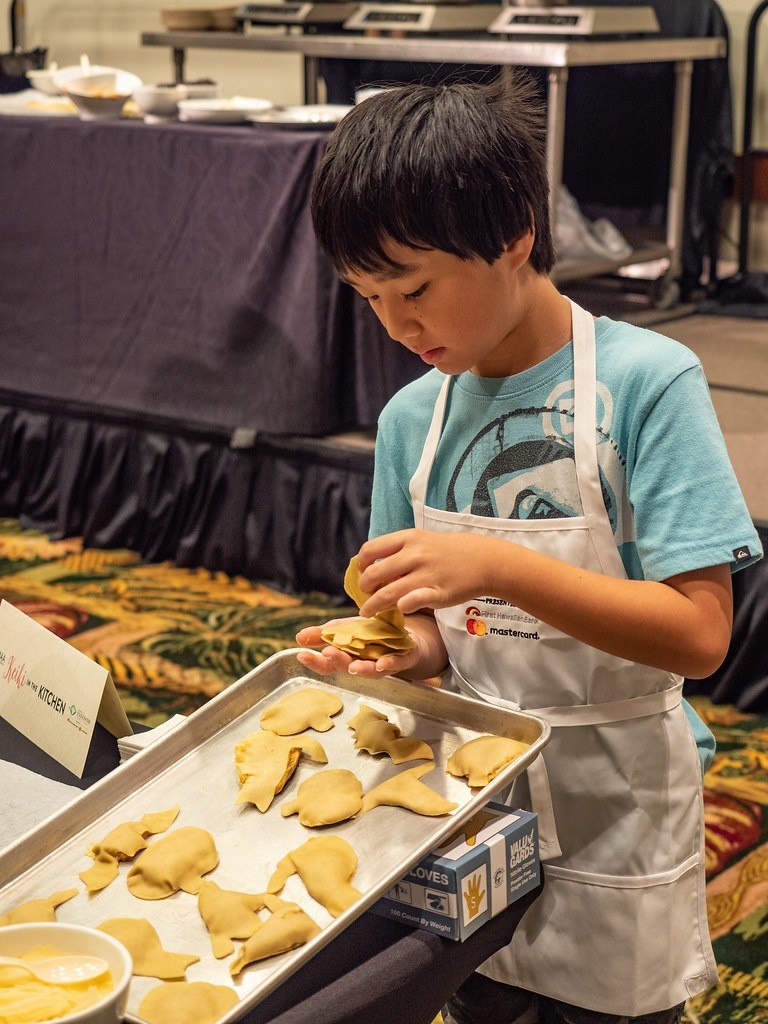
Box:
[0,71,430,441]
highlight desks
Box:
[141,23,730,310]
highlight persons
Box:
[295,80,764,1024]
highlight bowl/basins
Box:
[132,84,215,125]
[54,63,144,126]
[1,919,134,1024]
[26,69,63,95]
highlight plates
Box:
[244,99,359,129]
[179,98,269,124]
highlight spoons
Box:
[2,955,107,984]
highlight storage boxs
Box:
[366,800,541,944]
